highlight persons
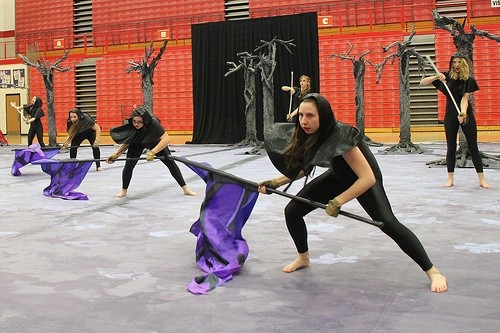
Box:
[419,53,490,188]
[282,75,311,124]
[257,93,447,293]
[14,96,46,148]
[60,108,103,171]
[106,107,197,197]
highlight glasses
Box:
[132,120,144,125]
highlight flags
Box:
[32,158,93,200]
[179,156,260,295]
[10,143,63,177]
